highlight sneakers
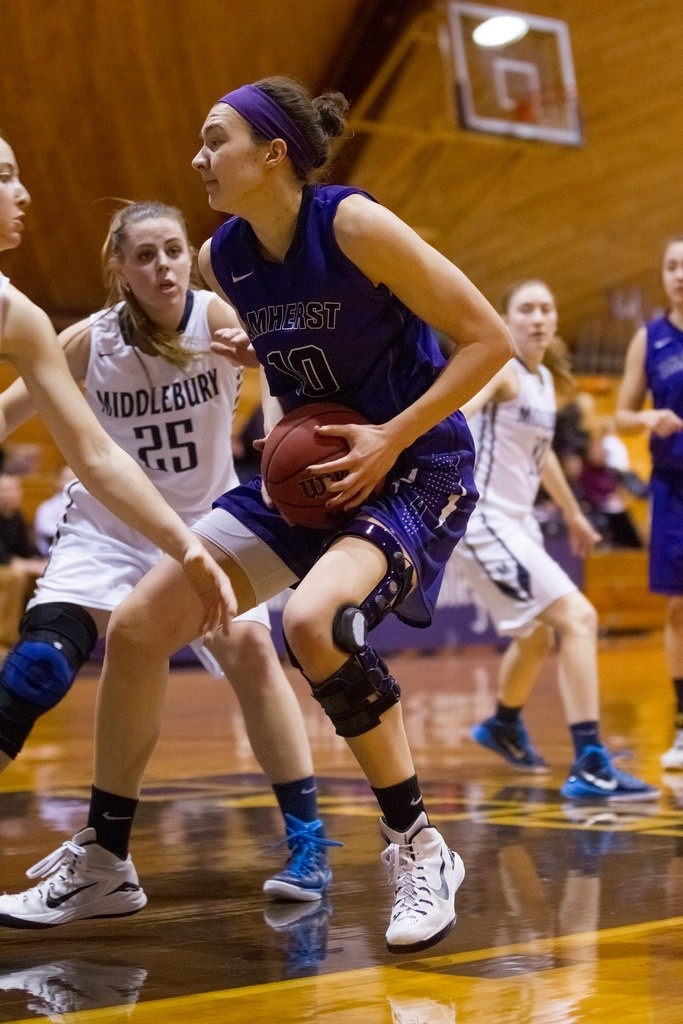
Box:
[560,745,662,802]
[263,814,344,901]
[379,811,465,955]
[662,731,683,769]
[472,714,551,773]
[387,986,455,1024]
[0,827,147,924]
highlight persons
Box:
[0,139,238,636]
[451,275,664,803]
[615,240,683,772]
[0,200,333,899]
[0,472,48,566]
[534,390,650,551]
[1,73,518,953]
[231,398,267,481]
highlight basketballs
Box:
[261,402,369,527]
